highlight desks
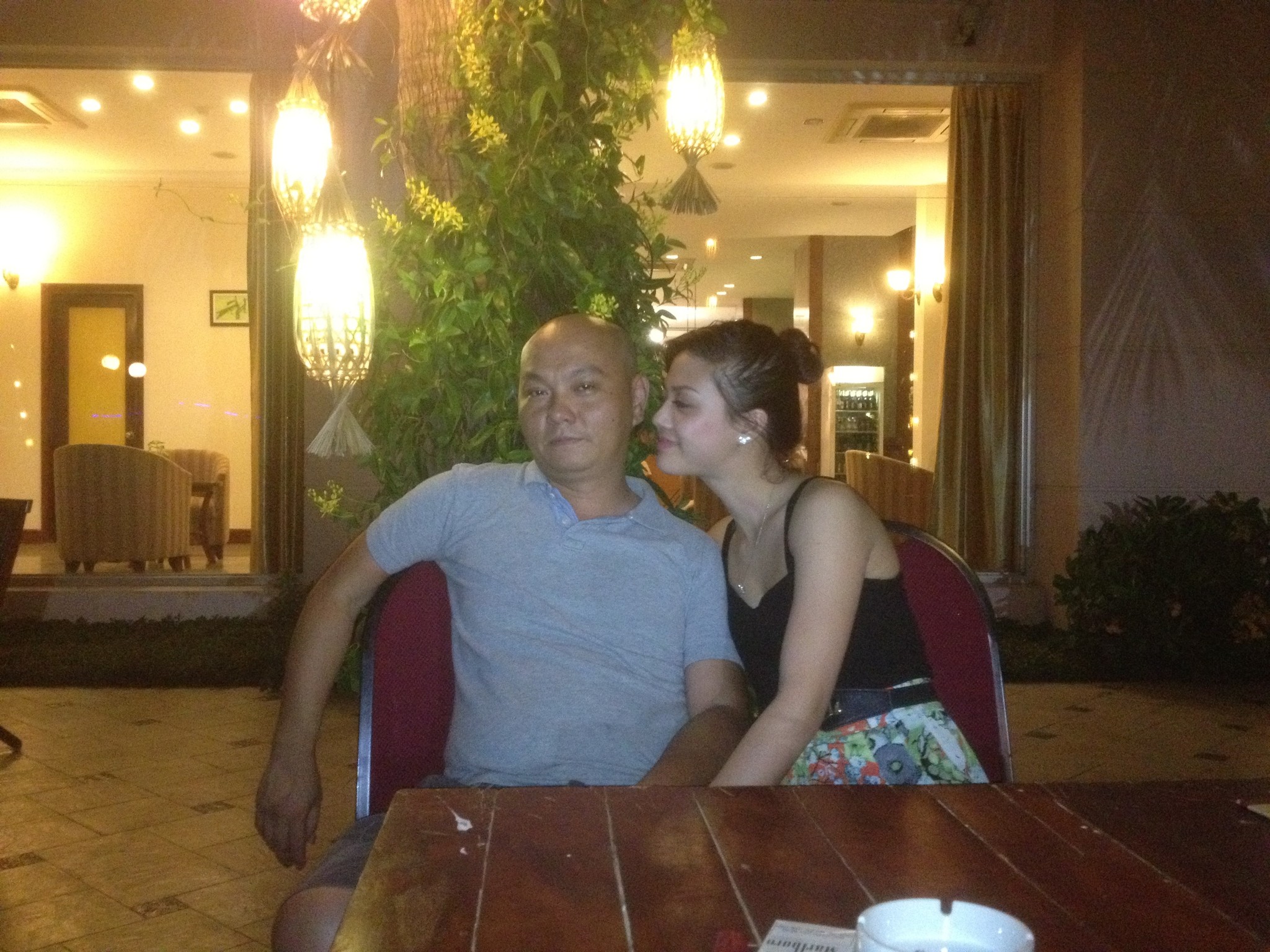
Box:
[191,482,219,564]
[326,778,1270,952]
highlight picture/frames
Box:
[210,290,249,327]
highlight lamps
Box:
[657,14,726,213]
[852,308,875,346]
[292,0,375,77]
[887,270,921,305]
[292,155,377,459]
[271,42,335,230]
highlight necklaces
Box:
[736,479,778,593]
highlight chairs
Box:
[356,552,456,820]
[880,520,1012,783]
[147,449,229,560]
[843,449,934,528]
[0,498,41,749]
[53,444,193,573]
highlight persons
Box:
[254,313,753,952]
[652,320,990,786]
